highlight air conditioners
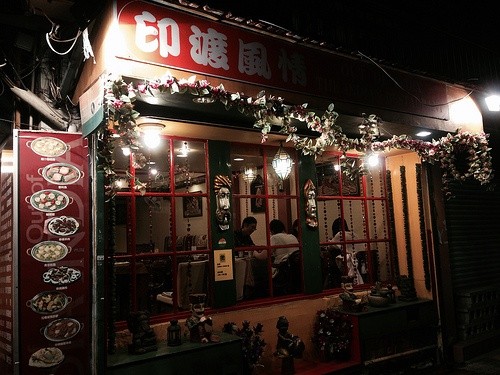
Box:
[271,139,294,181]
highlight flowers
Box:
[223,322,266,367]
[99,74,496,203]
[312,303,357,354]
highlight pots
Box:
[26,137,71,158]
[25,189,73,213]
[25,290,72,316]
[26,241,72,263]
[38,163,85,185]
[39,316,85,344]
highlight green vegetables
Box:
[48,167,73,182]
[32,193,65,210]
[33,295,66,312]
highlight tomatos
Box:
[41,199,48,204]
[51,199,55,204]
[44,191,51,195]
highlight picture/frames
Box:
[316,157,360,200]
[183,191,202,217]
[250,179,268,213]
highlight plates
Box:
[41,266,82,286]
[47,215,80,237]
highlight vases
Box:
[320,349,351,364]
[244,364,256,375]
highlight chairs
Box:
[156,259,209,312]
[287,250,379,295]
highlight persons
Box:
[251,219,300,298]
[273,316,299,359]
[326,217,365,254]
[232,217,257,256]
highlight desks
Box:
[336,297,436,360]
[112,261,147,322]
[230,245,339,303]
[178,257,253,301]
[109,330,244,375]
